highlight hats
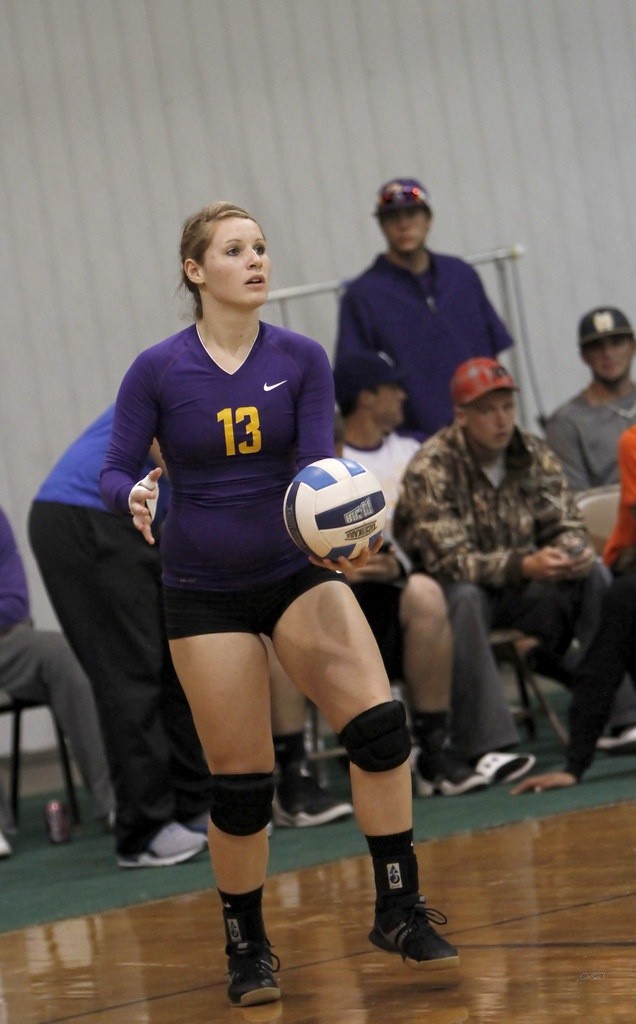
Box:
[452,356,519,405]
[579,307,633,345]
[335,350,412,389]
[373,178,432,218]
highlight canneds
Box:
[44,797,74,845]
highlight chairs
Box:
[0,484,636,839]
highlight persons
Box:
[0,179,636,869]
[99,202,460,1007]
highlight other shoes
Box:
[106,810,119,833]
[594,722,636,754]
[0,831,12,859]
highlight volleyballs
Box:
[283,457,386,563]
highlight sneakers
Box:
[269,773,354,825]
[225,940,281,1006]
[118,813,207,867]
[412,744,535,796]
[369,892,461,971]
